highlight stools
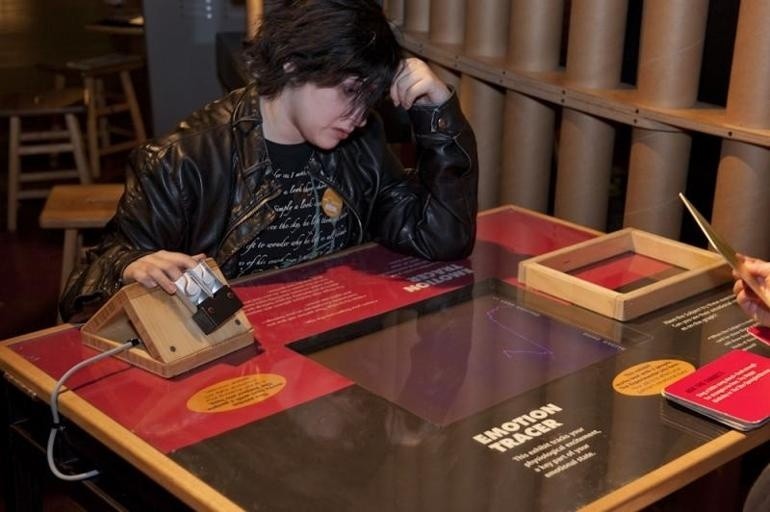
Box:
[2,84,95,235]
[39,181,128,325]
[49,51,149,183]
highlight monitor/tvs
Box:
[285,275,653,431]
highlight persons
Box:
[731,252,770,330]
[60,0,479,325]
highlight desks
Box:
[1,202,770,511]
